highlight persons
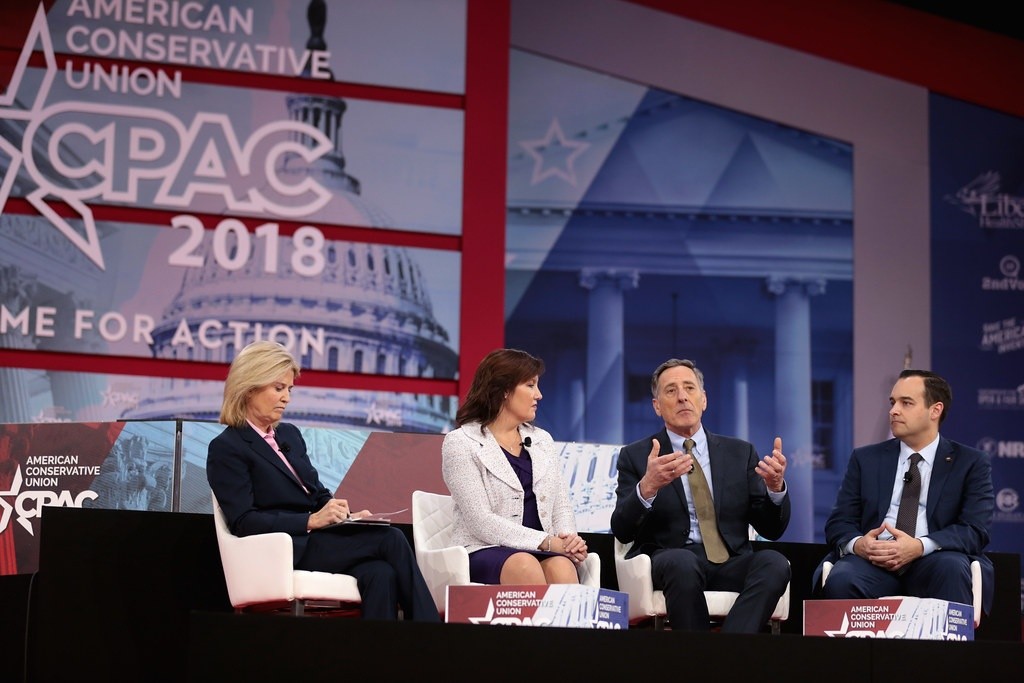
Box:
[610,358,793,634]
[811,370,996,616]
[206,339,443,621]
[442,348,589,585]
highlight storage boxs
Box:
[803,597,975,642]
[446,584,627,630]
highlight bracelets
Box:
[544,536,551,552]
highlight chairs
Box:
[209,489,361,616]
[820,558,982,642]
[612,536,792,635]
[410,490,599,624]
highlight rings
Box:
[672,470,675,474]
[582,540,586,544]
[340,513,343,517]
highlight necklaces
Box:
[498,440,517,451]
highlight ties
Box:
[683,439,729,564]
[893,452,924,541]
